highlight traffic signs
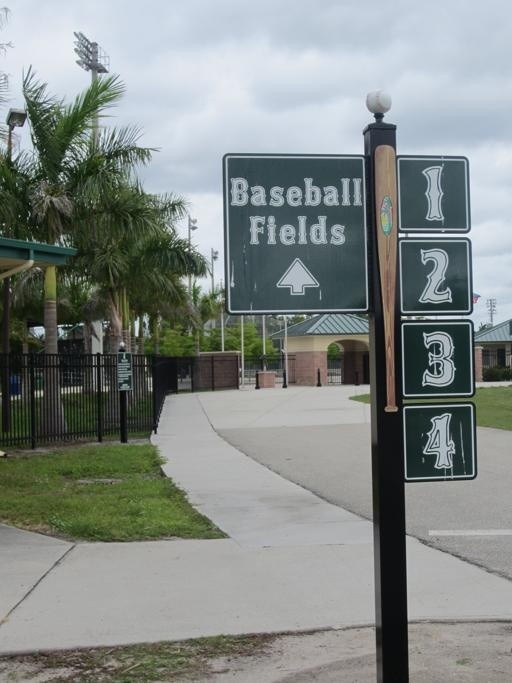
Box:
[224,154,370,313]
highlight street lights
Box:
[210,250,219,296]
[487,298,497,326]
[4,106,28,161]
[184,213,198,333]
